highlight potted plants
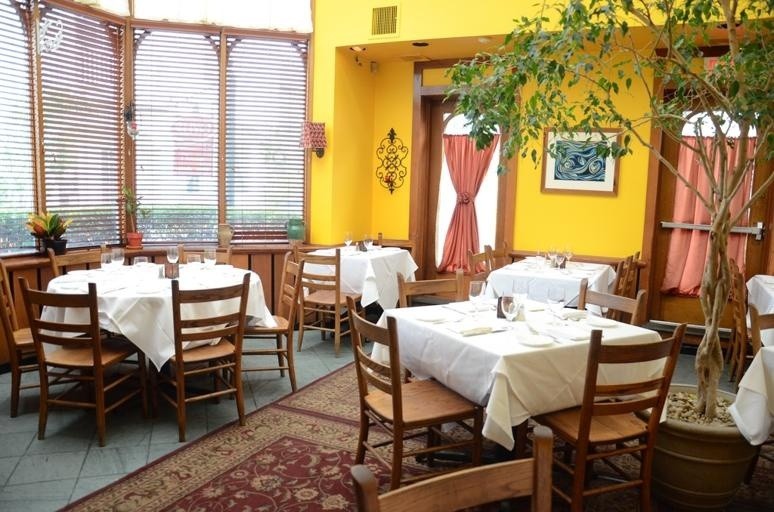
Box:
[113,184,155,250]
[442,0,772,510]
[25,209,75,257]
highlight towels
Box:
[560,326,604,341]
[552,307,587,321]
[445,321,492,336]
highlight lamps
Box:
[301,120,328,158]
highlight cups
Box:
[548,250,557,266]
[501,295,520,330]
[186,254,201,265]
[204,249,216,266]
[134,257,148,268]
[112,248,125,267]
[512,279,530,299]
[536,249,547,262]
[363,234,373,251]
[343,233,353,247]
[166,247,180,264]
[548,286,566,327]
[101,253,112,269]
[566,248,574,262]
[555,255,566,270]
[468,281,486,300]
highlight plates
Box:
[418,316,446,321]
[520,336,553,346]
[591,318,618,328]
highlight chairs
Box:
[46,243,109,277]
[724,258,750,365]
[514,323,686,510]
[342,297,488,494]
[378,238,416,261]
[396,268,467,447]
[220,249,305,401]
[292,245,366,357]
[467,245,491,290]
[174,243,234,269]
[0,258,92,418]
[728,267,762,394]
[743,303,774,487]
[602,255,634,322]
[18,276,151,448]
[621,252,642,321]
[347,425,554,512]
[149,272,251,442]
[578,277,650,327]
[487,241,509,271]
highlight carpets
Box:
[56,353,774,512]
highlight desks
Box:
[34,262,279,379]
[739,273,774,348]
[368,290,676,464]
[297,245,419,323]
[478,254,617,318]
[728,343,774,448]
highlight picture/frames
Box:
[537,123,622,199]
